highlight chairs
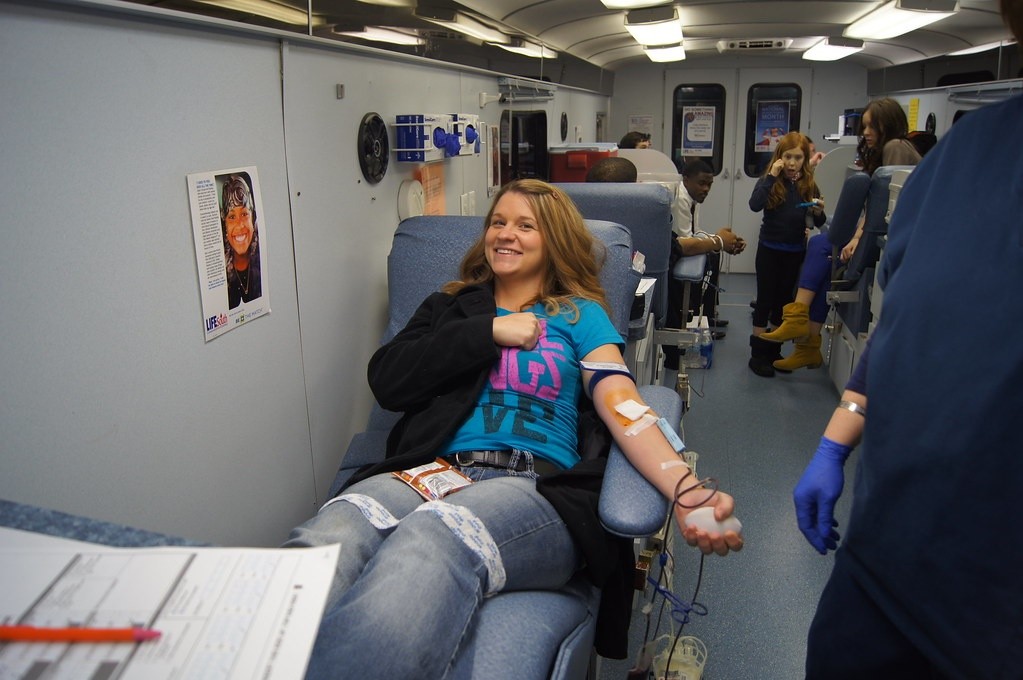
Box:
[325,145,918,680]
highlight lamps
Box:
[841,0,961,40]
[623,5,684,46]
[643,41,686,63]
[802,37,865,61]
[412,4,513,45]
[486,38,558,59]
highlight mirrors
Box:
[123,0,615,97]
[867,38,1023,96]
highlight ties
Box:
[691,203,695,234]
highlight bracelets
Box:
[840,400,865,416]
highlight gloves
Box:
[793,436,854,555]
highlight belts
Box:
[444,450,558,475]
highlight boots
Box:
[750,328,791,377]
[773,333,822,370]
[759,302,809,343]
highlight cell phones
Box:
[796,202,819,209]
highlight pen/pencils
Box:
[0,626,161,642]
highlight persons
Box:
[619,132,651,149]
[805,136,825,166]
[747,132,827,378]
[757,127,786,145]
[282,179,743,680]
[221,176,262,310]
[587,158,737,301]
[671,161,746,340]
[785,0,1023,680]
[760,100,921,370]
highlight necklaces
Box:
[234,264,249,294]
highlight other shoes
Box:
[711,331,725,338]
[708,317,728,326]
